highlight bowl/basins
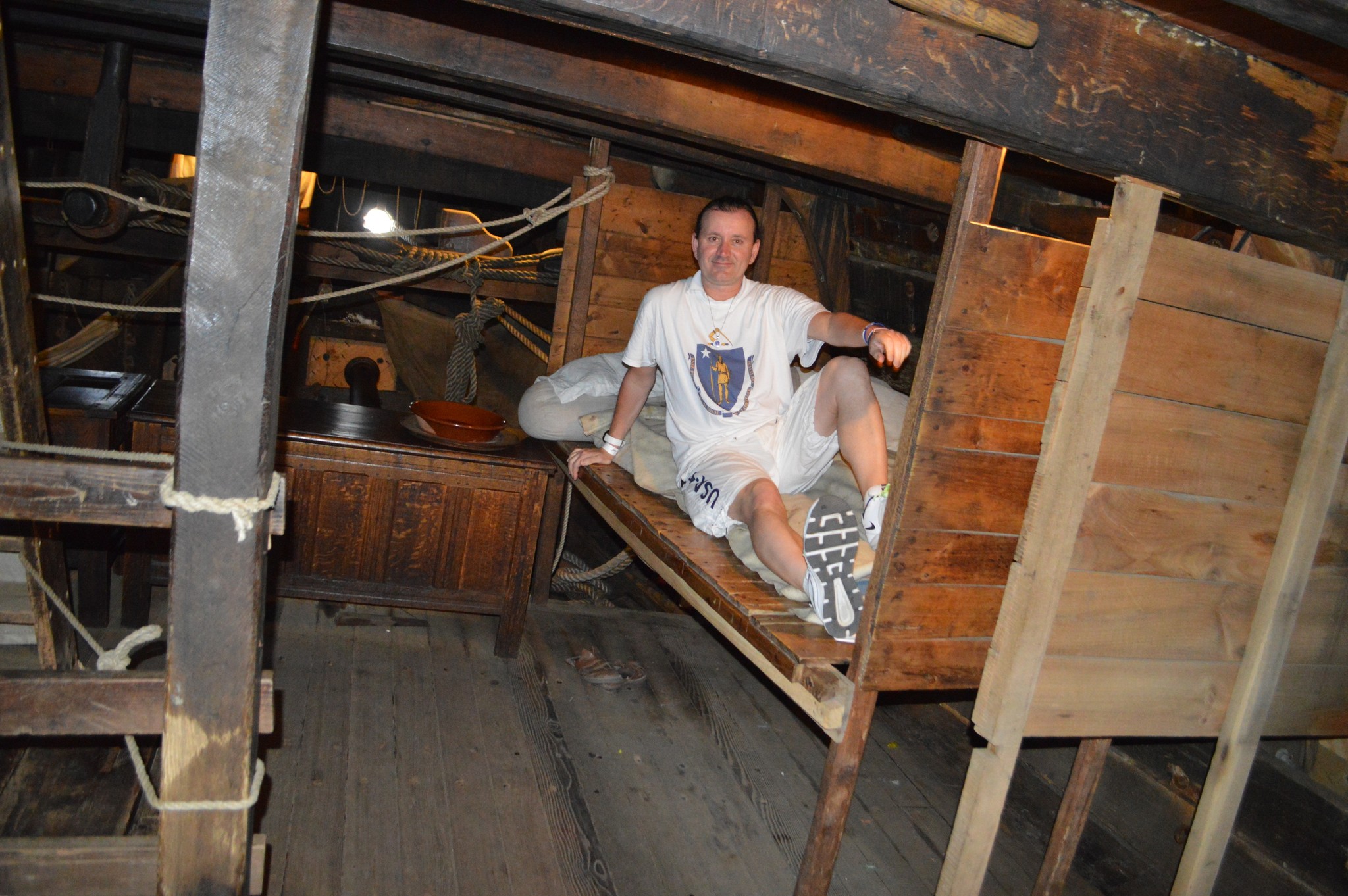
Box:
[409,400,506,443]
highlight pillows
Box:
[535,349,665,405]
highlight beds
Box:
[533,135,1112,896]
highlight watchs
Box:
[603,431,626,449]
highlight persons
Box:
[565,196,915,640]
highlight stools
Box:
[304,320,397,391]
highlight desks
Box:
[39,368,150,627]
[116,378,558,656]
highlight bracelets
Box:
[602,442,618,456]
[861,321,885,345]
[867,326,889,348]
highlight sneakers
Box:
[861,484,889,552]
[804,495,864,638]
